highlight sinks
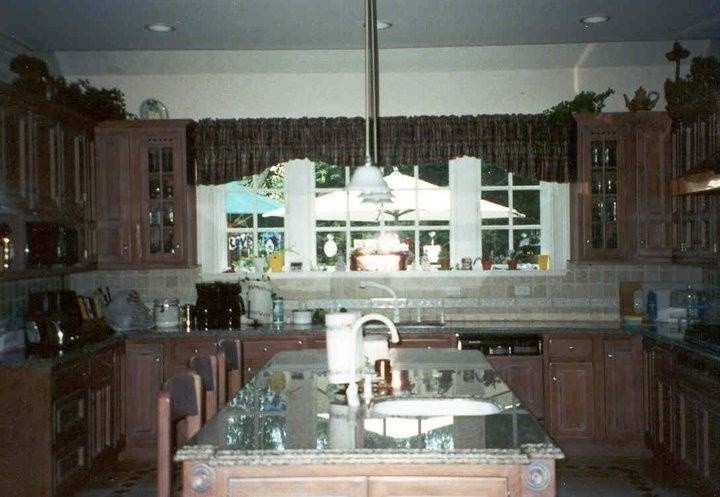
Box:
[369,395,503,416]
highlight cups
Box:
[537,254,549,271]
[273,296,284,325]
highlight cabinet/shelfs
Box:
[602,328,650,456]
[241,332,307,386]
[490,355,541,421]
[645,333,719,495]
[0,83,91,283]
[568,110,671,263]
[543,327,602,455]
[91,119,198,268]
[0,340,120,497]
[670,96,719,269]
[119,333,167,460]
[308,329,455,350]
[169,332,215,446]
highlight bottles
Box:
[147,146,176,254]
[591,144,616,221]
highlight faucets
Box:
[345,312,401,408]
[359,280,400,324]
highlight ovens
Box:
[24,307,84,355]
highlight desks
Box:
[175,347,566,495]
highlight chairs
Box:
[185,352,220,438]
[214,337,245,406]
[152,373,210,496]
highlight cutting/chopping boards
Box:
[618,280,654,324]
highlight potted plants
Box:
[498,252,524,269]
[473,255,493,270]
[437,246,450,268]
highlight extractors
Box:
[670,149,720,196]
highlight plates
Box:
[140,99,168,121]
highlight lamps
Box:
[344,0,395,204]
